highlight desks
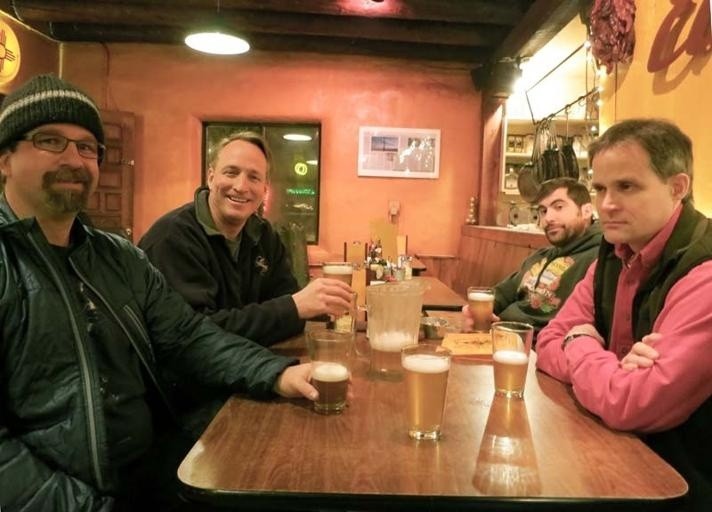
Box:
[416,254,458,279]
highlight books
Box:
[439,331,526,364]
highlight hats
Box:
[1,74,105,167]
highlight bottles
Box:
[506,132,598,154]
[401,256,413,279]
[506,168,519,188]
[365,239,393,323]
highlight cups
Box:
[354,279,432,376]
[398,342,452,444]
[304,327,358,416]
[467,286,496,333]
[322,259,355,320]
[492,320,533,402]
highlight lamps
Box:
[282,128,315,142]
[184,1,252,55]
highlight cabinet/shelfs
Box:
[500,117,602,194]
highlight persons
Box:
[460,176,604,346]
[137,131,355,443]
[535,116,711,510]
[0,72,355,512]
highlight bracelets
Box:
[560,333,601,353]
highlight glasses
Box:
[13,132,106,160]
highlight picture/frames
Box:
[355,125,441,181]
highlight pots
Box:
[516,110,580,204]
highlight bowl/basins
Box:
[420,316,448,339]
